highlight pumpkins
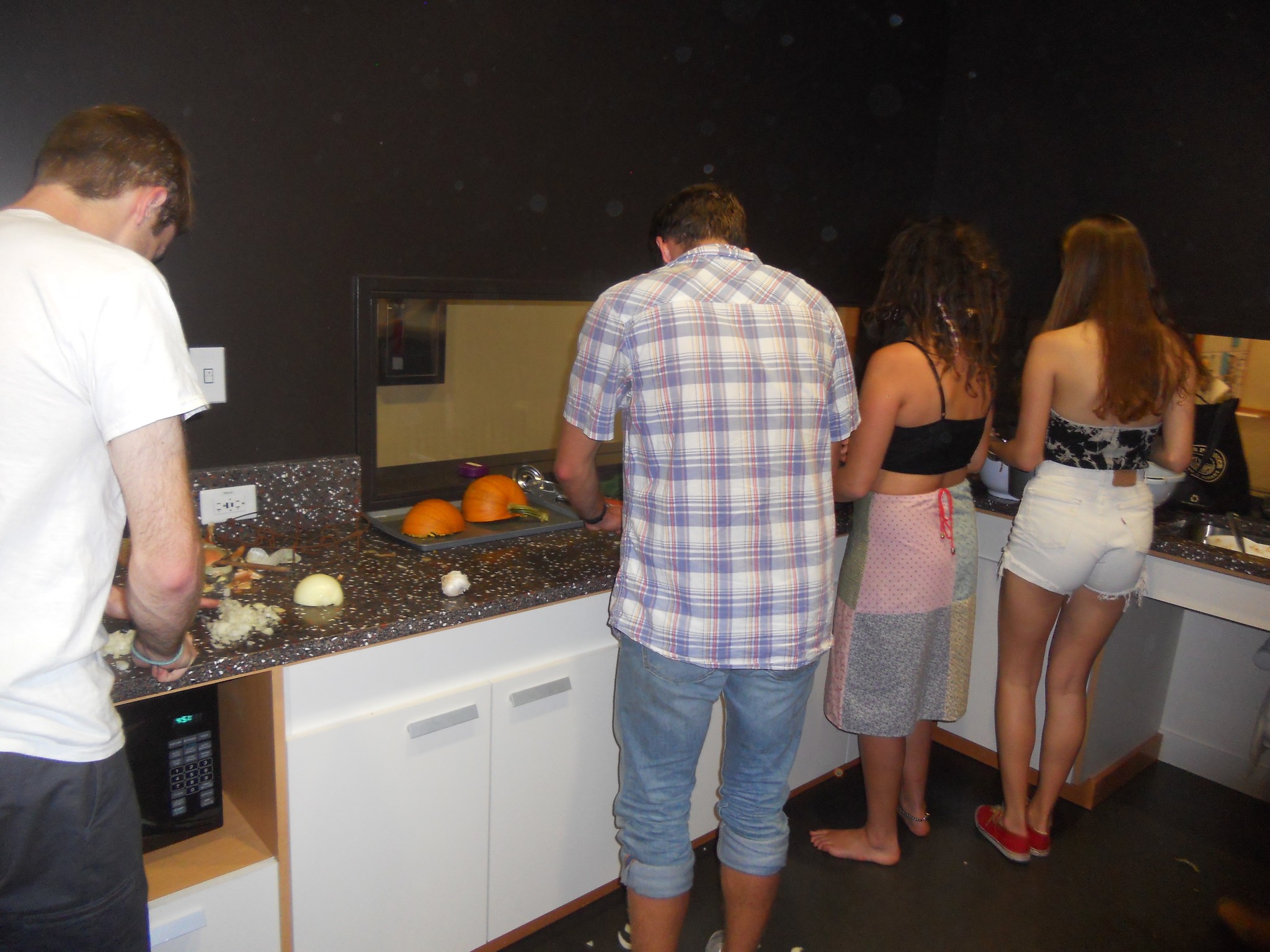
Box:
[461,475,550,522]
[294,573,344,606]
[402,499,464,537]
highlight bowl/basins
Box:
[1139,459,1186,510]
[977,440,1027,502]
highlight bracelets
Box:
[584,503,607,525]
[131,635,184,666]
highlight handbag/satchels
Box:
[1163,395,1251,515]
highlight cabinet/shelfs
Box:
[146,555,1074,952]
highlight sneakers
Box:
[1025,821,1050,856]
[974,804,1031,861]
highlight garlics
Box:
[442,571,470,597]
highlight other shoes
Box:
[705,930,724,952]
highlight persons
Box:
[974,211,1194,862]
[556,183,860,952]
[0,104,202,952]
[812,220,1004,864]
